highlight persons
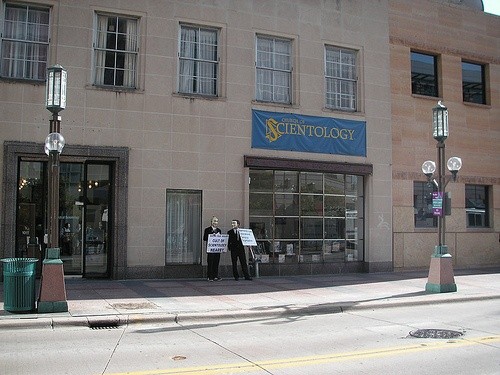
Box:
[203,216,223,283]
[226,220,253,281]
[60,224,81,256]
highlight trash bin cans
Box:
[0,258,40,312]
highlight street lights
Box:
[421,99,463,294]
[37,64,70,314]
